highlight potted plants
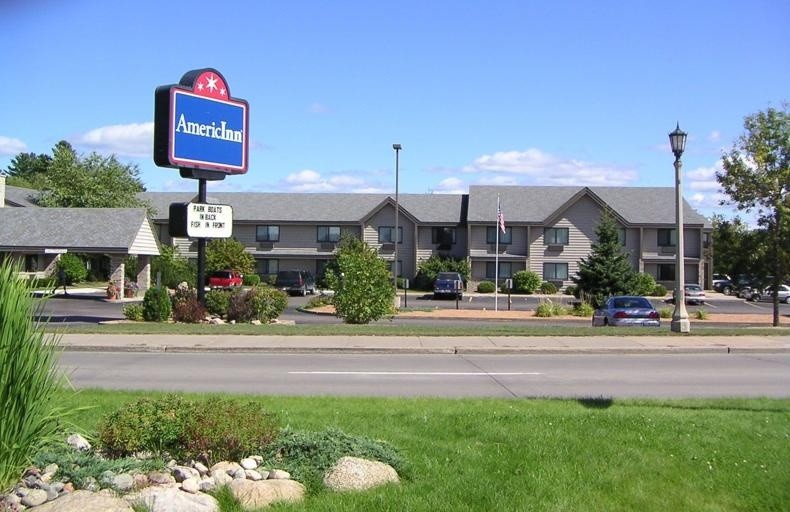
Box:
[107,279,139,299]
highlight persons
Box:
[49,265,69,297]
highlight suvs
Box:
[276,268,316,297]
[431,271,463,299]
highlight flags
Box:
[498,201,506,234]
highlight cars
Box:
[671,283,706,305]
[592,295,661,328]
[209,269,243,290]
[712,273,790,305]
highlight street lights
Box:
[392,144,403,293]
[668,119,691,333]
